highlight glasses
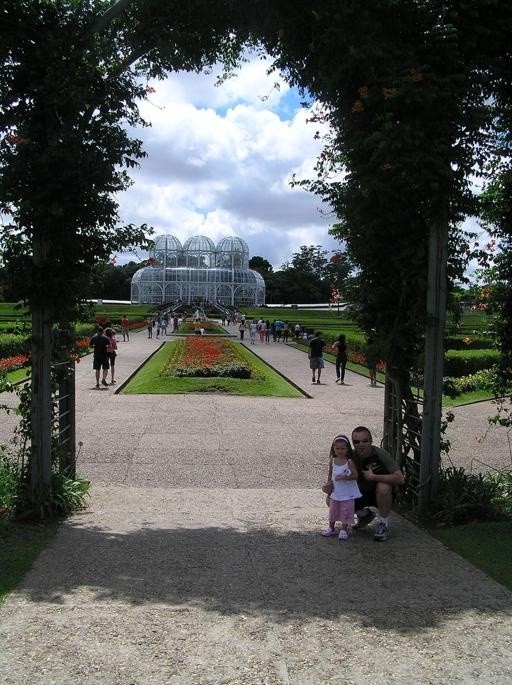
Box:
[354,439,370,443]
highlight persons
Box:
[332,335,349,384]
[88,305,303,387]
[321,435,363,540]
[322,426,405,541]
[308,333,326,383]
[366,338,376,385]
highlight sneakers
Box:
[337,529,349,540]
[311,376,321,385]
[350,507,376,531]
[95,379,116,389]
[320,526,336,536]
[373,521,391,542]
[368,383,376,387]
[335,379,344,385]
